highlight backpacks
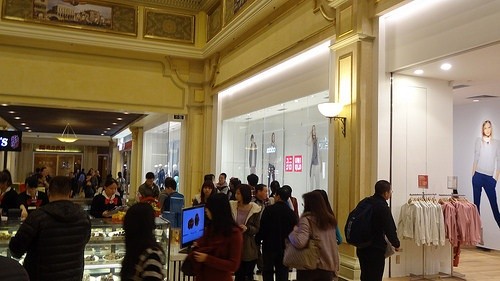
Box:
[80,172,86,183]
[344,197,388,246]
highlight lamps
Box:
[317,102,347,140]
[57,122,79,143]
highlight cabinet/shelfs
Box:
[0,214,173,281]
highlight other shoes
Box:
[252,268,292,274]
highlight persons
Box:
[69,168,101,198]
[230,184,261,281]
[356,180,403,281]
[267,133,276,186]
[306,125,322,189]
[472,121,500,227]
[289,190,343,281]
[255,185,300,281]
[120,202,166,281]
[0,256,30,281]
[192,173,241,206]
[192,192,242,281]
[138,172,159,201]
[8,175,92,281]
[115,172,125,198]
[0,167,51,220]
[90,178,124,219]
[159,177,185,227]
[249,135,257,174]
[247,174,280,275]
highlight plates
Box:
[81,230,125,281]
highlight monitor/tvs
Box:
[180,204,206,246]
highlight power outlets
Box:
[396,255,401,264]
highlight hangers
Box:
[407,192,470,206]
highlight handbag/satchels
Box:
[181,245,218,276]
[283,215,321,270]
